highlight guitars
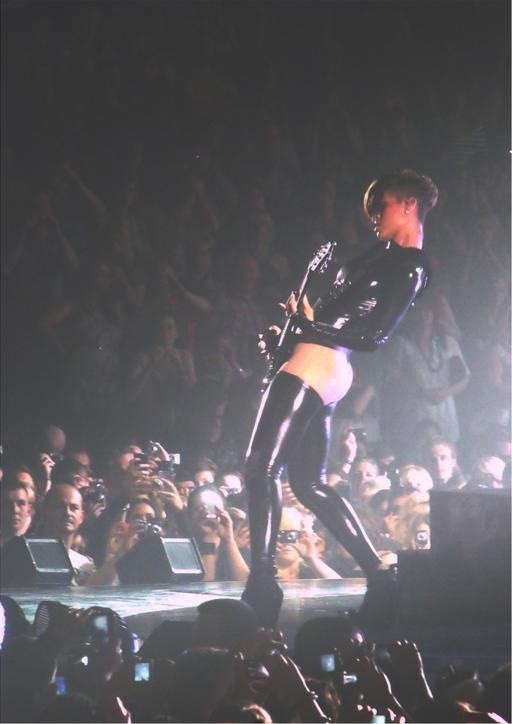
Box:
[260,240,338,392]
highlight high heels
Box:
[351,564,399,624]
[240,576,285,629]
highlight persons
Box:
[1,121,511,724]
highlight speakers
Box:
[114,535,205,583]
[0,534,78,585]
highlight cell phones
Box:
[134,452,148,474]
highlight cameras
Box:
[277,529,298,544]
[348,428,366,441]
[150,470,176,491]
[199,504,219,521]
[415,529,428,547]
[133,519,162,537]
[74,613,371,693]
[168,453,181,465]
[90,482,109,508]
